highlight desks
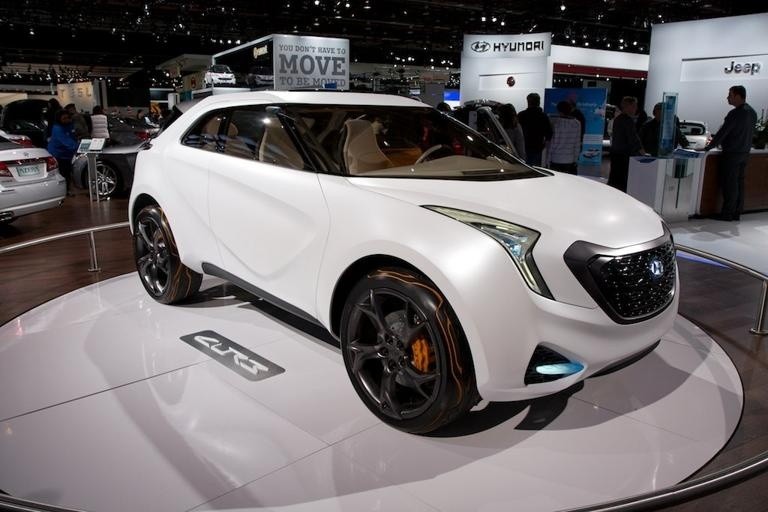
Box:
[681,144,768,215]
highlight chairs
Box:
[338,119,395,176]
[256,118,307,171]
[205,117,256,161]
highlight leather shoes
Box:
[710,213,740,221]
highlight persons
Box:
[437,102,451,111]
[497,93,586,177]
[159,104,170,117]
[88,105,110,138]
[640,102,690,155]
[44,98,66,142]
[47,111,79,199]
[137,109,146,122]
[65,103,89,136]
[694,85,757,221]
[606,95,653,194]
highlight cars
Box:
[0,141,67,224]
[582,147,600,158]
[204,65,236,88]
[606,104,621,140]
[242,66,273,86]
[675,119,714,151]
[0,100,203,201]
[128,91,679,436]
